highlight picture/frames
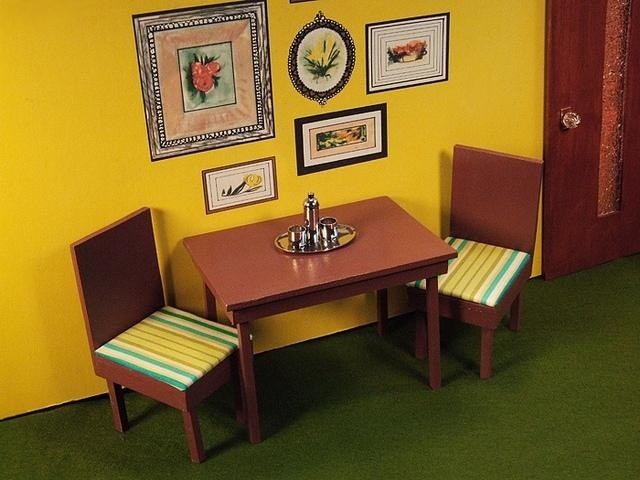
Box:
[202,156,279,215]
[294,102,387,176]
[288,10,357,106]
[365,11,451,94]
[132,0,274,161]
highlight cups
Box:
[319,217,339,248]
[286,224,307,249]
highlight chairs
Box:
[71,206,249,463]
[404,143,545,380]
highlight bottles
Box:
[303,192,321,250]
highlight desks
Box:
[183,196,459,445]
